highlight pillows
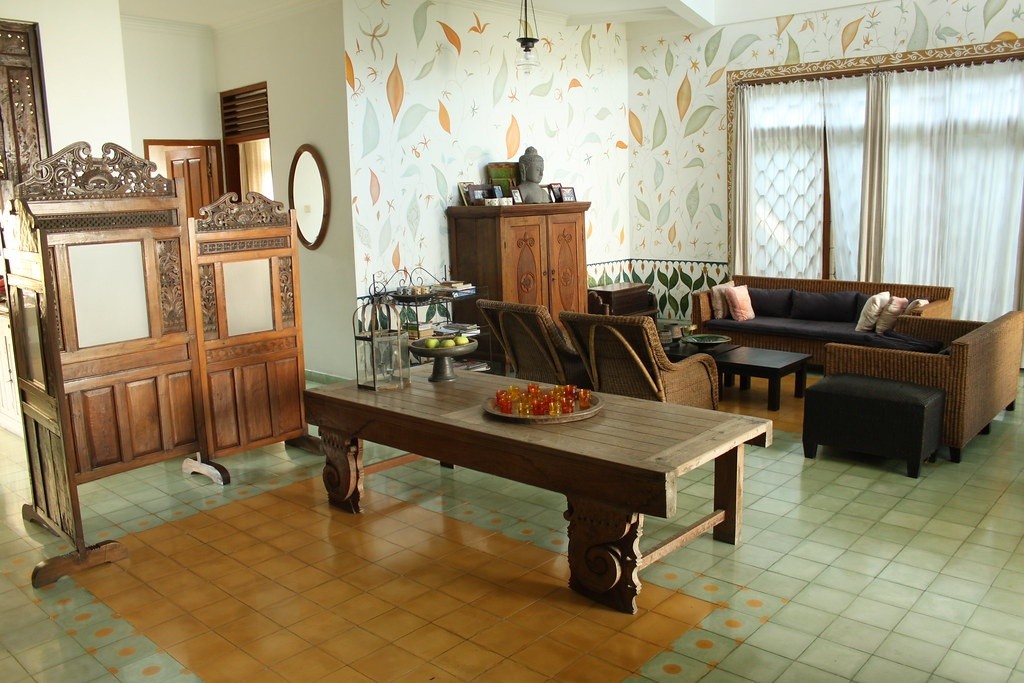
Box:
[877,295,908,334]
[727,285,755,320]
[905,299,929,314]
[711,281,734,318]
[855,292,890,333]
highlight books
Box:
[434,328,459,335]
[402,321,434,340]
[432,281,476,298]
[442,323,480,337]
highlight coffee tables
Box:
[659,336,814,411]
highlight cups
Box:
[485,197,512,206]
[496,382,592,415]
[396,286,429,295]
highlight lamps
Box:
[514,0,542,80]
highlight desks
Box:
[589,283,659,326]
[303,364,773,615]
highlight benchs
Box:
[820,311,1024,463]
[692,274,953,364]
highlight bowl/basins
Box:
[681,334,732,350]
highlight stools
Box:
[802,374,944,479]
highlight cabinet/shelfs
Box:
[380,269,479,354]
[447,201,588,315]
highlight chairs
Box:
[558,311,720,411]
[475,299,589,389]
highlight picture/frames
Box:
[467,184,493,205]
[550,182,562,202]
[510,186,524,205]
[559,187,576,202]
[458,182,474,206]
[492,184,504,198]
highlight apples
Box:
[425,336,469,348]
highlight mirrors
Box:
[288,143,330,251]
